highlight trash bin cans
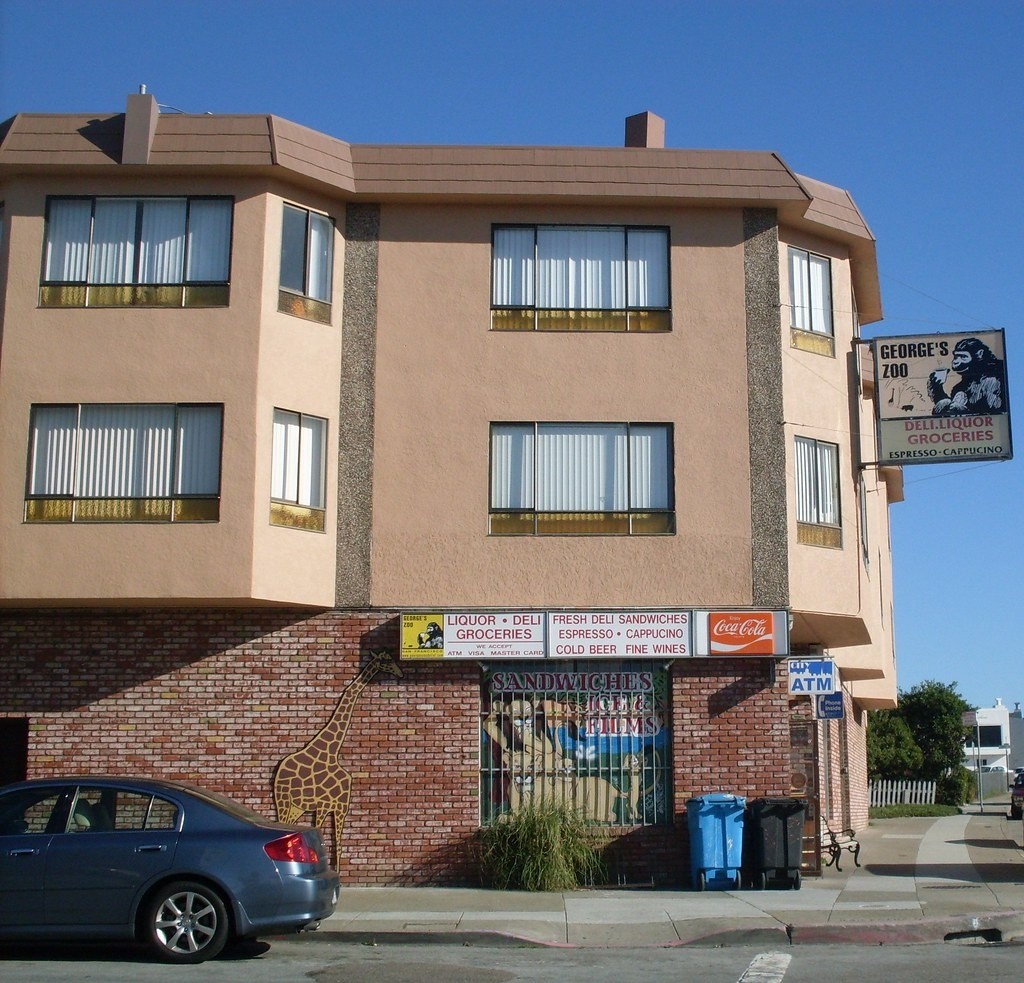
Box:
[683,793,748,887]
[748,792,811,888]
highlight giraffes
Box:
[273,651,405,887]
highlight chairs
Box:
[92,802,113,832]
[73,798,97,832]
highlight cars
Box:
[974,765,1008,773]
[0,775,341,964]
[1013,767,1024,781]
[1009,770,1024,820]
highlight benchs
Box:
[820,815,861,872]
